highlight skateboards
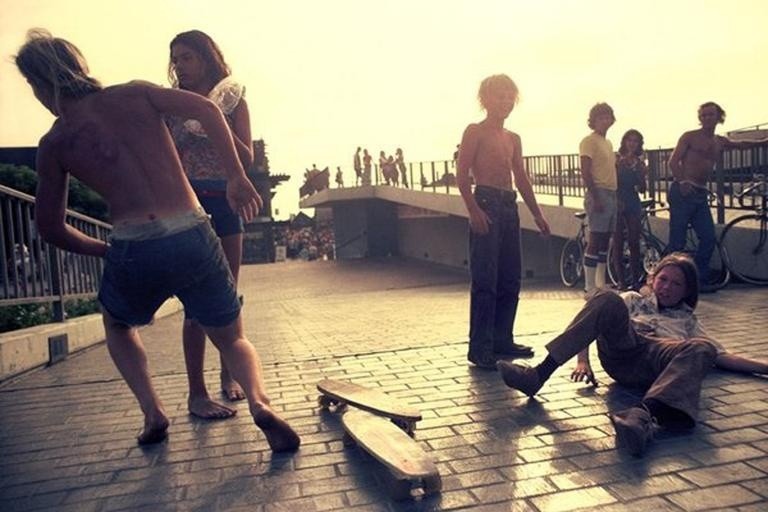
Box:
[315,378,422,435]
[340,410,441,499]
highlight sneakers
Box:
[493,339,533,356]
[468,348,513,369]
[610,406,661,459]
[494,360,542,396]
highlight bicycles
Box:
[559,179,768,292]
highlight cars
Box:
[6,241,39,281]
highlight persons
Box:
[160,30,254,418]
[581,103,618,294]
[13,28,300,451]
[456,75,550,369]
[274,144,410,261]
[496,252,768,459]
[658,101,768,293]
[613,129,646,291]
